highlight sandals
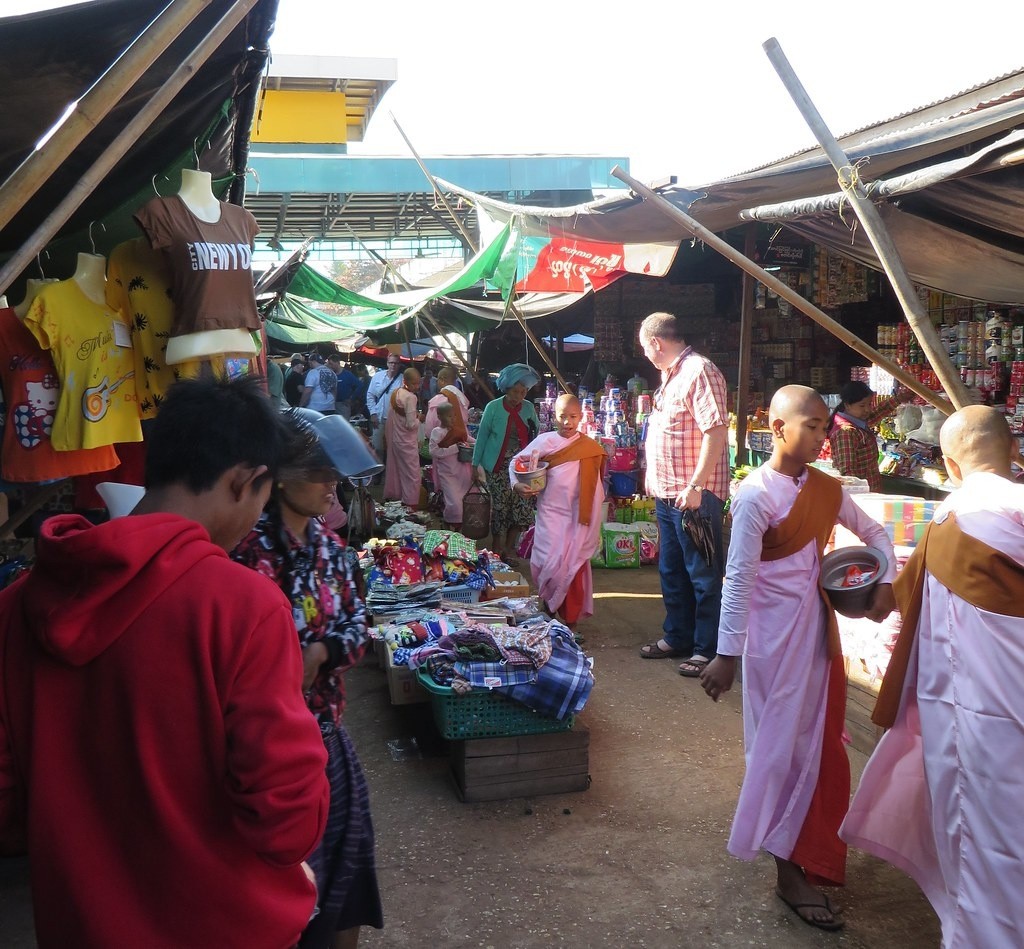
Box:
[678,655,713,676]
[640,641,693,658]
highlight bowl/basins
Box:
[512,462,549,491]
[459,444,474,462]
[820,546,889,619]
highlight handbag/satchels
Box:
[360,396,380,417]
[462,479,491,539]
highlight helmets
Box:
[276,407,349,467]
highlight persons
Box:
[837,405,1024,949]
[418,367,441,416]
[699,385,897,933]
[827,380,914,492]
[508,394,608,644]
[366,352,405,485]
[282,351,371,421]
[0,373,331,948]
[425,367,470,492]
[639,312,731,677]
[228,406,385,949]
[428,403,483,532]
[470,364,539,567]
[382,368,424,511]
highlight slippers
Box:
[500,556,520,567]
[775,883,846,930]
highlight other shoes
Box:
[574,632,584,643]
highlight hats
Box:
[290,359,306,367]
[309,353,325,364]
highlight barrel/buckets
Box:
[609,469,638,496]
[608,446,637,470]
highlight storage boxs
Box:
[362,553,575,737]
[593,279,738,367]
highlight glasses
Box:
[436,377,441,383]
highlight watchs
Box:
[689,482,704,493]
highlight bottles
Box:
[957,362,1024,434]
[600,374,636,457]
[877,322,944,392]
[546,383,564,398]
[878,434,946,484]
[632,382,655,444]
[643,495,656,521]
[616,499,631,523]
[864,392,898,451]
[468,408,484,423]
[534,398,558,435]
[418,410,425,442]
[936,311,1024,361]
[578,386,606,459]
[628,374,648,392]
[728,408,770,430]
[468,425,479,439]
